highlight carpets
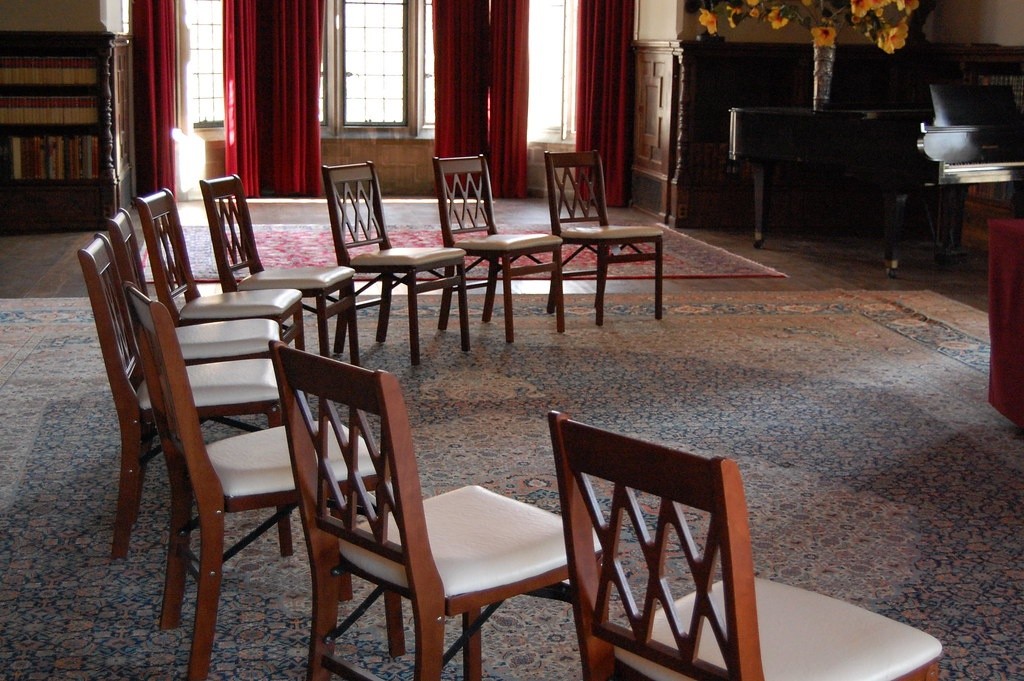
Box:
[137,223,787,285]
[0,288,1023,680]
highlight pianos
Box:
[727,82,1024,277]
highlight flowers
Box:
[698,0,921,56]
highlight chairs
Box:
[549,408,946,681]
[107,206,285,365]
[135,186,305,352]
[541,149,666,328]
[123,280,406,681]
[77,232,294,561]
[321,161,470,364]
[430,153,566,343]
[198,172,358,365]
[266,338,604,681]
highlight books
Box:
[0,57,98,178]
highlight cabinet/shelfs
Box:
[0,30,121,235]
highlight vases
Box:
[810,44,836,112]
